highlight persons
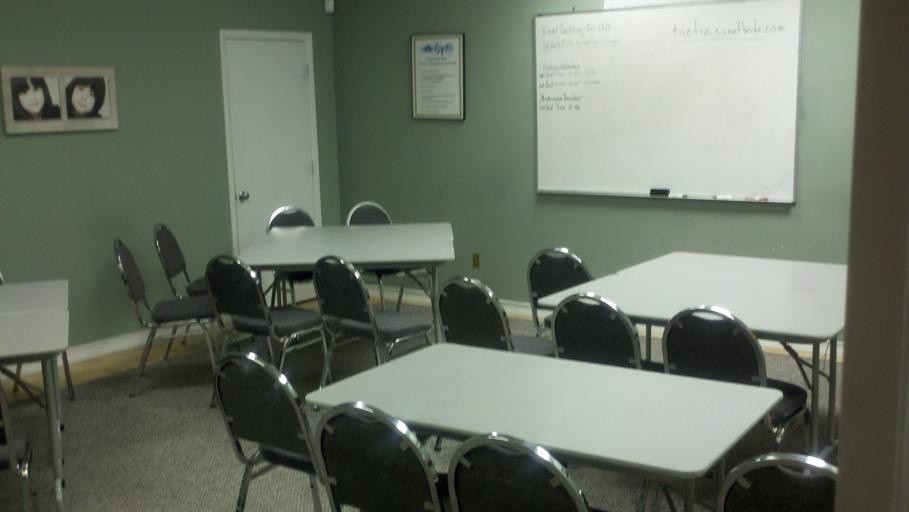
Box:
[64,76,106,119]
[10,76,61,121]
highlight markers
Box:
[676,195,768,201]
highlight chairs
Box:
[0,386,34,511]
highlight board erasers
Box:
[649,189,670,197]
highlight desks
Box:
[0,279,76,512]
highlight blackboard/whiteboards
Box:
[534,6,799,205]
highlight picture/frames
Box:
[0,65,119,137]
[411,33,466,121]
